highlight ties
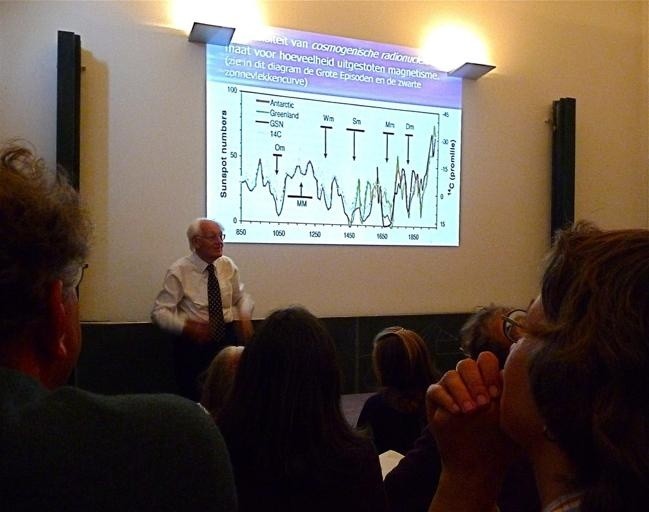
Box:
[207,265,226,343]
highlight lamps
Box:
[449,60,495,79]
[188,21,235,46]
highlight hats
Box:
[500,308,541,343]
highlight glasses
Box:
[199,234,226,241]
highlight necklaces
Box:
[543,483,589,512]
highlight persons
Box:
[149,217,257,401]
[230,306,388,511]
[202,346,246,424]
[424,217,649,512]
[358,324,442,455]
[0,135,237,510]
[384,302,525,510]
